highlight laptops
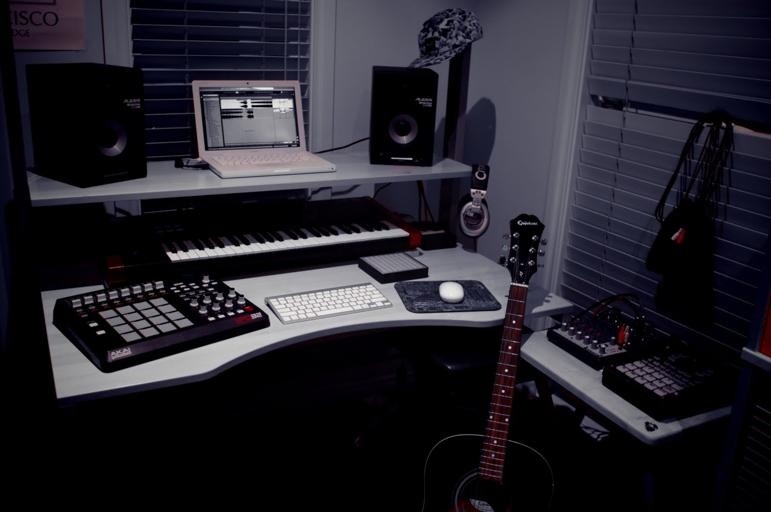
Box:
[191,79,336,179]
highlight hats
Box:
[408,7,485,68]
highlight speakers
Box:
[26,61,147,189]
[369,65,439,167]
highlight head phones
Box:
[458,162,491,238]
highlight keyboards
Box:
[264,282,392,324]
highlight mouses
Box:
[439,281,465,302]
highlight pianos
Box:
[114,196,410,283]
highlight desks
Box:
[703,341,770,511]
[26,146,578,408]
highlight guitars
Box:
[421,214,554,511]
